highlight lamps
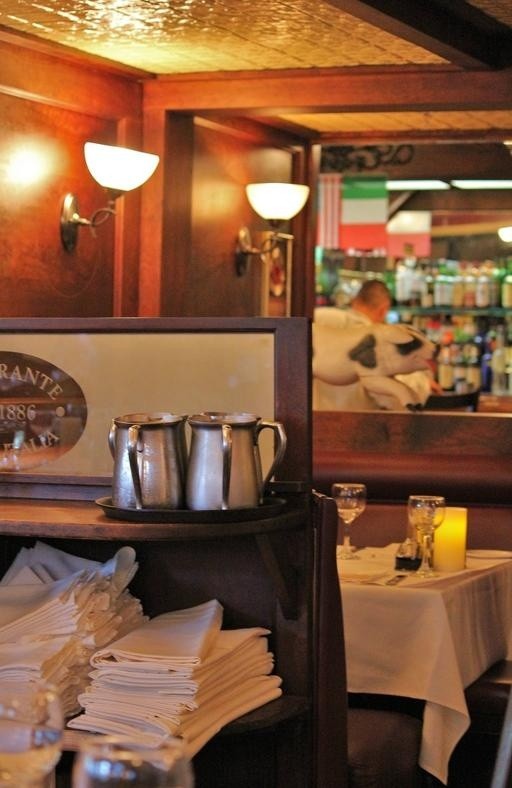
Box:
[56,139,161,253]
[229,179,310,276]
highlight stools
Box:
[461,658,512,705]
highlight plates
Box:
[336,562,389,579]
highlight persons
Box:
[310,279,443,411]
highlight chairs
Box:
[310,485,422,786]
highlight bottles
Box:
[392,257,512,309]
[422,322,511,398]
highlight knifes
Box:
[386,575,408,586]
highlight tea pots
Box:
[107,409,286,510]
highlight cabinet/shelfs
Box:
[380,300,511,393]
[4,494,305,736]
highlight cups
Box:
[434,506,467,574]
[67,734,196,788]
[1,687,66,787]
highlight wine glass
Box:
[332,483,369,558]
[406,495,446,579]
